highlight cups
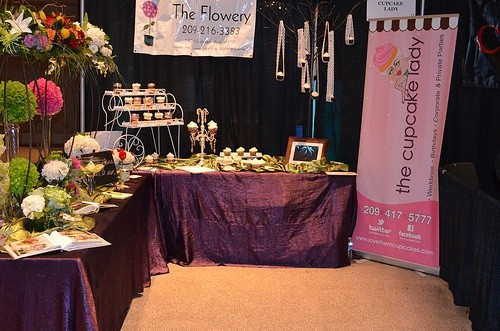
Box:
[164,112,172,124]
[132,83,141,93]
[156,97,165,104]
[134,97,141,109]
[131,114,139,126]
[124,97,133,108]
[148,83,155,94]
[120,169,130,182]
[143,97,153,108]
[144,113,152,121]
[154,113,163,120]
[113,83,122,94]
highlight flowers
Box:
[21,134,105,220]
[0,4,113,78]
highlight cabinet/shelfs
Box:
[102,89,185,158]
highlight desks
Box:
[0,158,358,331]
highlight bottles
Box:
[348,237,353,260]
[295,112,304,139]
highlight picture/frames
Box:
[285,136,329,165]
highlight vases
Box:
[24,218,61,232]
